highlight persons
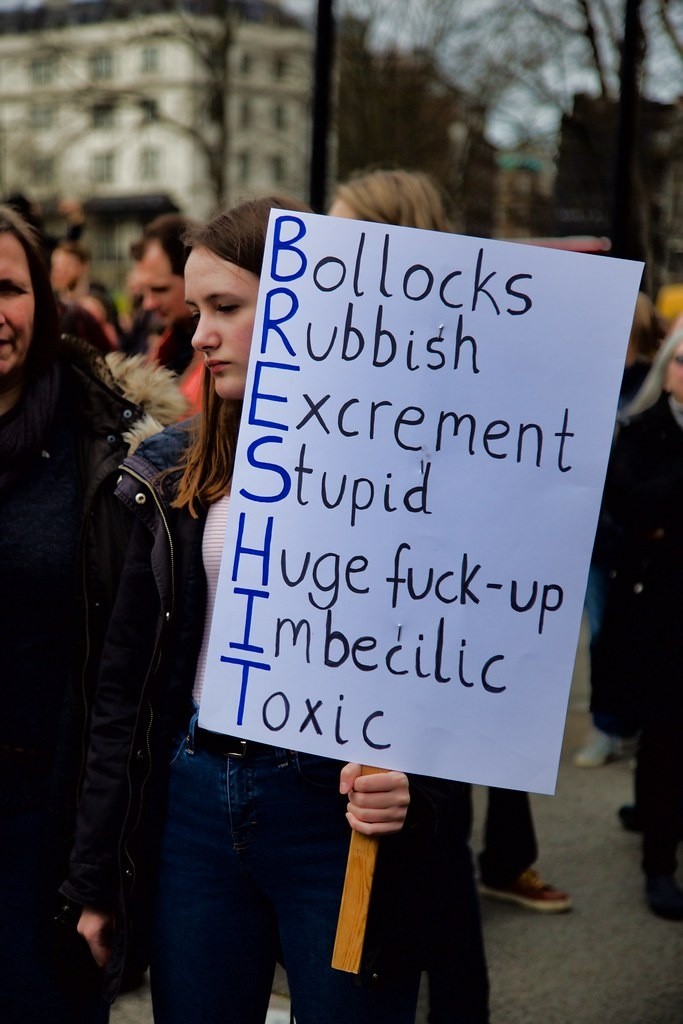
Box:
[61,195,428,1023]
[0,169,682,1024]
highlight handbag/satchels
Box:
[588,578,655,740]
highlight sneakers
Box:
[478,867,572,913]
